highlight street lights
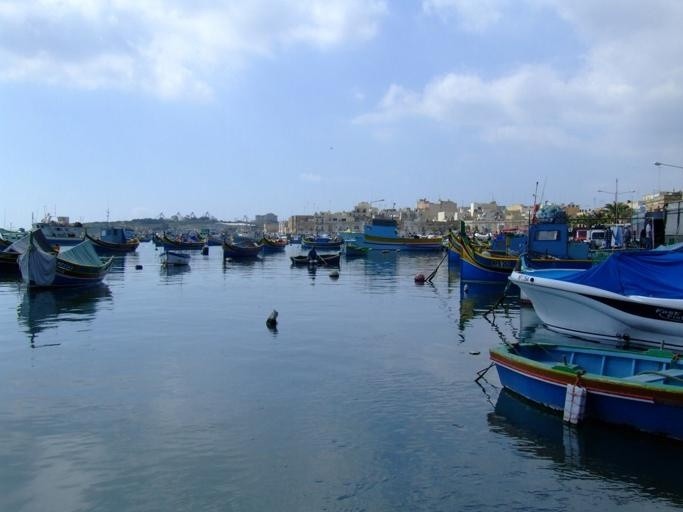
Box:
[652,161,683,168]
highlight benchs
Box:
[621,368,683,382]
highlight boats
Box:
[508,242,683,356]
[0,231,115,288]
[489,340,683,437]
[339,213,443,250]
[443,223,683,285]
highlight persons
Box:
[604,226,615,249]
[644,221,652,249]
[307,247,316,259]
[622,226,631,250]
